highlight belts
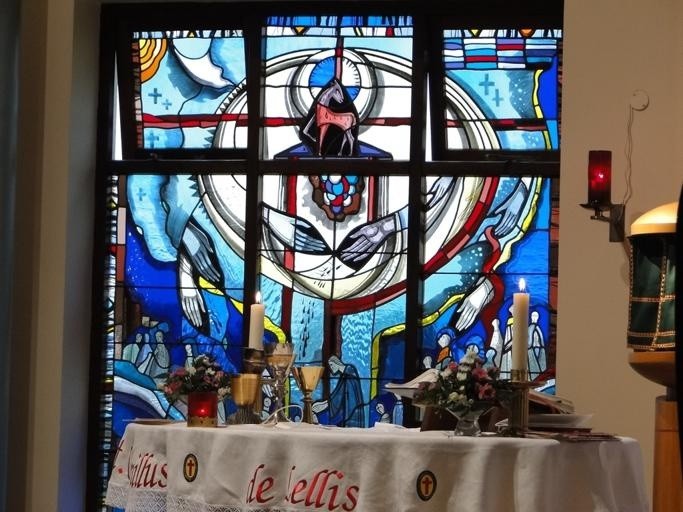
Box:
[264,343,326,425]
[229,373,261,425]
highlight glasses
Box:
[574,142,626,245]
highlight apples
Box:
[245,289,268,351]
[510,273,534,379]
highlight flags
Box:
[182,388,225,429]
[438,402,488,437]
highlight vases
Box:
[403,344,519,414]
[148,351,239,402]
